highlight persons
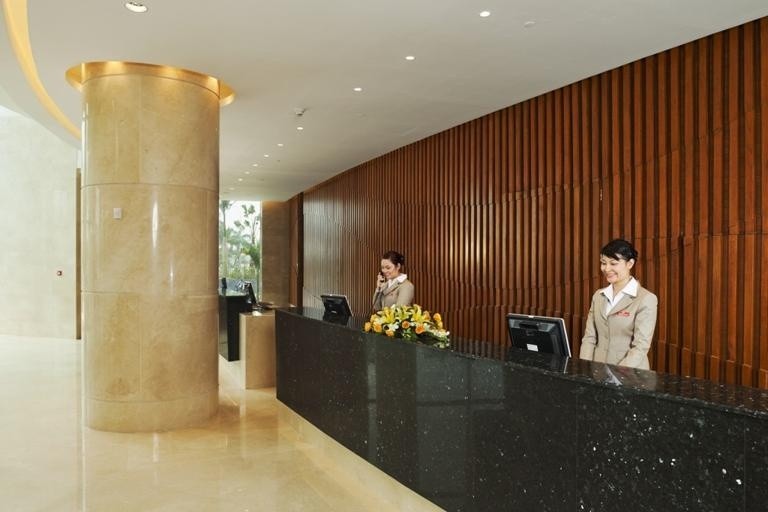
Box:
[370,250,418,335]
[577,237,661,371]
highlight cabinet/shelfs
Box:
[238,310,275,392]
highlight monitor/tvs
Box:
[244,281,256,304]
[320,294,354,317]
[505,313,572,358]
[221,277,227,289]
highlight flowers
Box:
[364,304,452,344]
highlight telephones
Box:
[379,270,385,283]
